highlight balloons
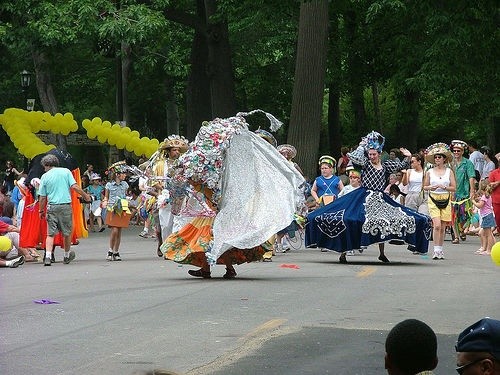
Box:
[0,236,11,252]
[491,242,500,266]
[0,108,165,159]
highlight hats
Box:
[452,140,467,150]
[14,177,27,196]
[345,165,362,178]
[90,173,102,180]
[277,143,296,159]
[255,129,277,148]
[160,135,188,153]
[455,318,500,353]
[425,142,454,163]
[107,161,128,174]
[318,156,337,167]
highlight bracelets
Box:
[39,210,44,213]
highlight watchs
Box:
[443,186,447,190]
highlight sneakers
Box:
[433,250,445,259]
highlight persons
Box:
[0,119,500,279]
[454,317,500,375]
[385,319,439,375]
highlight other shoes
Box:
[480,249,491,255]
[476,247,486,253]
[452,226,498,244]
[322,248,328,252]
[9,221,289,279]
[378,254,390,263]
[339,256,348,263]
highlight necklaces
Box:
[437,167,444,180]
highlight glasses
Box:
[456,357,491,375]
[410,160,419,164]
[453,149,463,153]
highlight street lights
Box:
[20,69,33,173]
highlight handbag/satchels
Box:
[429,192,450,208]
